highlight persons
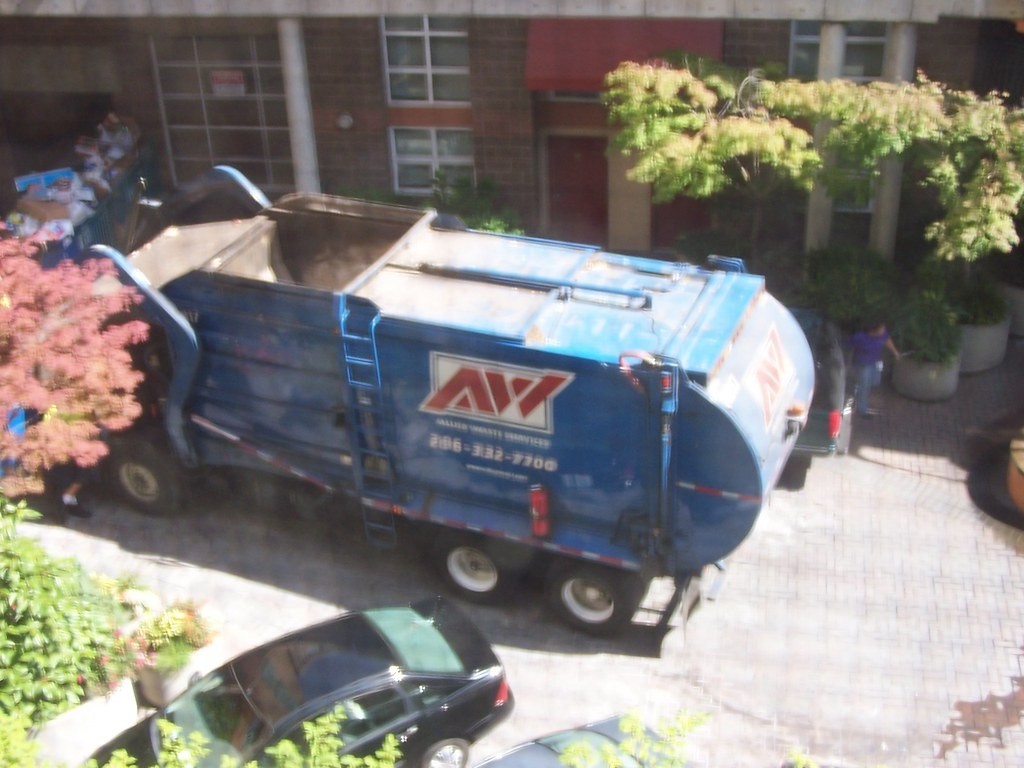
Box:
[846,317,900,421]
[42,384,95,515]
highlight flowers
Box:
[129,603,214,669]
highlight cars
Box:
[79,593,519,768]
[775,302,854,461]
[469,713,676,768]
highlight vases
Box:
[140,662,195,708]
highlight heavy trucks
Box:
[70,156,817,638]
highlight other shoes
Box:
[61,499,93,518]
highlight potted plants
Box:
[945,266,1011,372]
[893,287,962,401]
